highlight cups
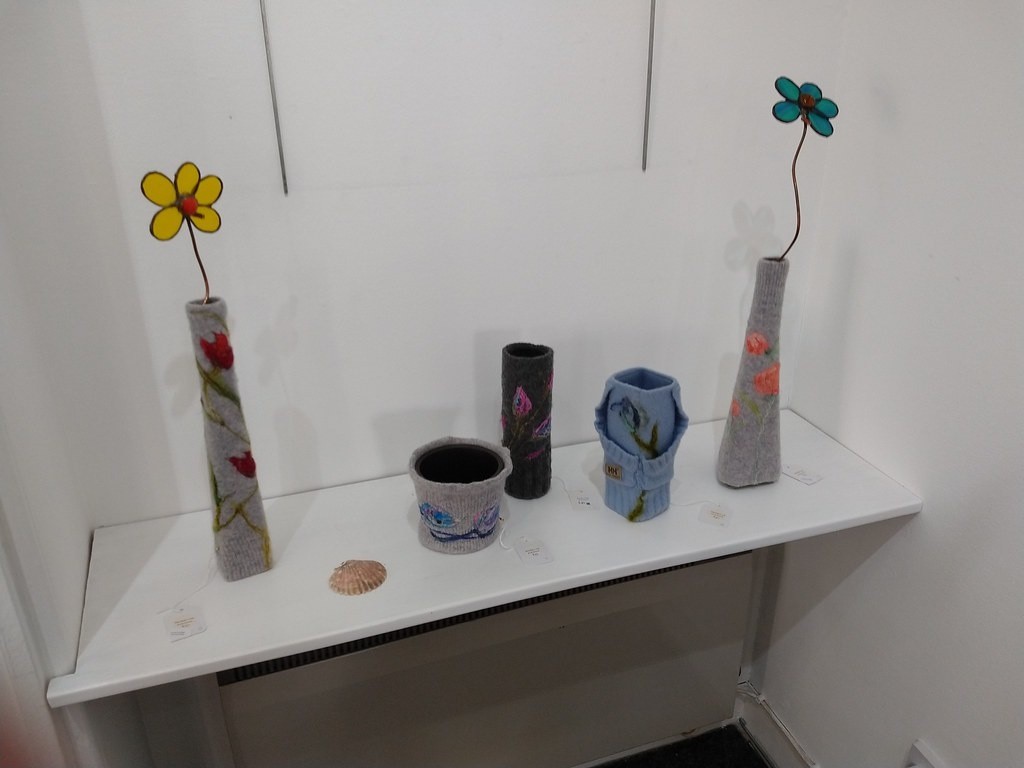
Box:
[501,342,555,501]
[594,366,689,523]
[408,436,512,555]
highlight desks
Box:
[44,407,923,768]
